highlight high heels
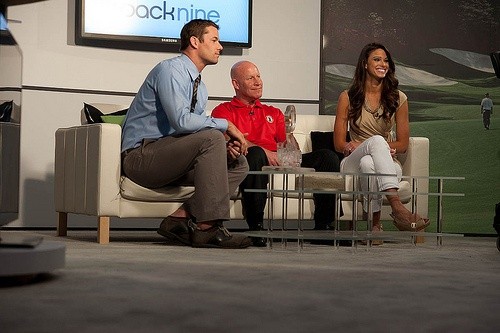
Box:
[389,214,430,232]
[372,225,383,244]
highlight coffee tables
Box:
[243,171,465,249]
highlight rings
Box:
[348,148,351,150]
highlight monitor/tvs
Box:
[74,0,252,47]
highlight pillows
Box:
[82,102,129,124]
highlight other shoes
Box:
[249,224,266,247]
[157,216,253,249]
[311,226,352,247]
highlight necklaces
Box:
[363,101,382,114]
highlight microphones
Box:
[250,111,254,115]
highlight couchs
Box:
[53,102,431,244]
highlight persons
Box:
[480,93,493,129]
[333,43,431,246]
[211,61,353,247]
[121,20,252,248]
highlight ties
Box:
[190,74,201,113]
